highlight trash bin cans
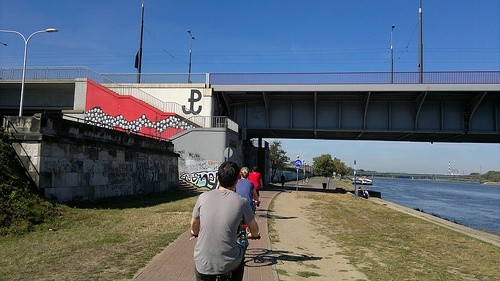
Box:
[322,183,327,189]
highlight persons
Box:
[235,167,261,214]
[358,187,369,199]
[191,161,260,281]
[246,166,263,207]
[281,174,285,187]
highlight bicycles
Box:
[188,231,261,281]
[237,200,262,261]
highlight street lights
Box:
[390,24,395,84]
[0,28,59,116]
[186,29,195,82]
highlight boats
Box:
[352,177,372,185]
